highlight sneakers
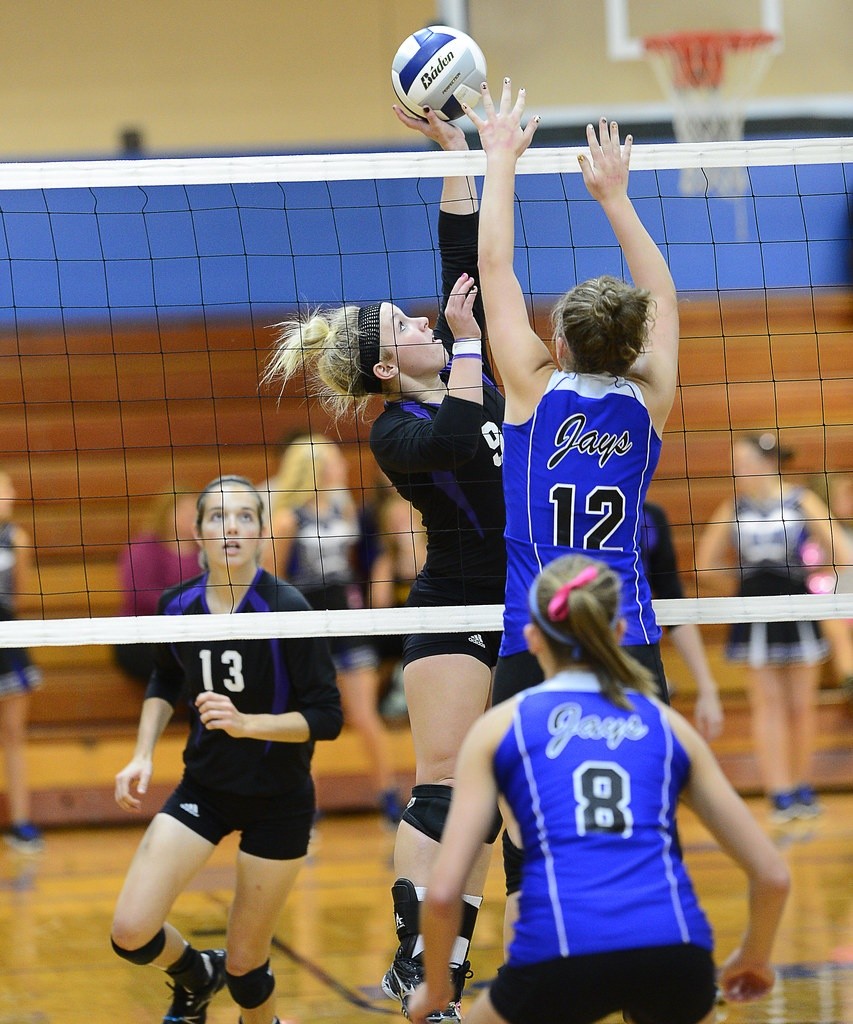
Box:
[382,877,481,1023]
[161,948,227,1024]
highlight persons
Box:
[457,78,680,965]
[271,75,508,1024]
[109,475,344,1024]
[690,430,853,839]
[404,554,793,1023]
[116,431,724,828]
[0,472,48,855]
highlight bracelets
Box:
[452,339,482,356]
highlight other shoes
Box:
[5,822,42,854]
[774,788,817,825]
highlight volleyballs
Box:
[390,24,488,125]
